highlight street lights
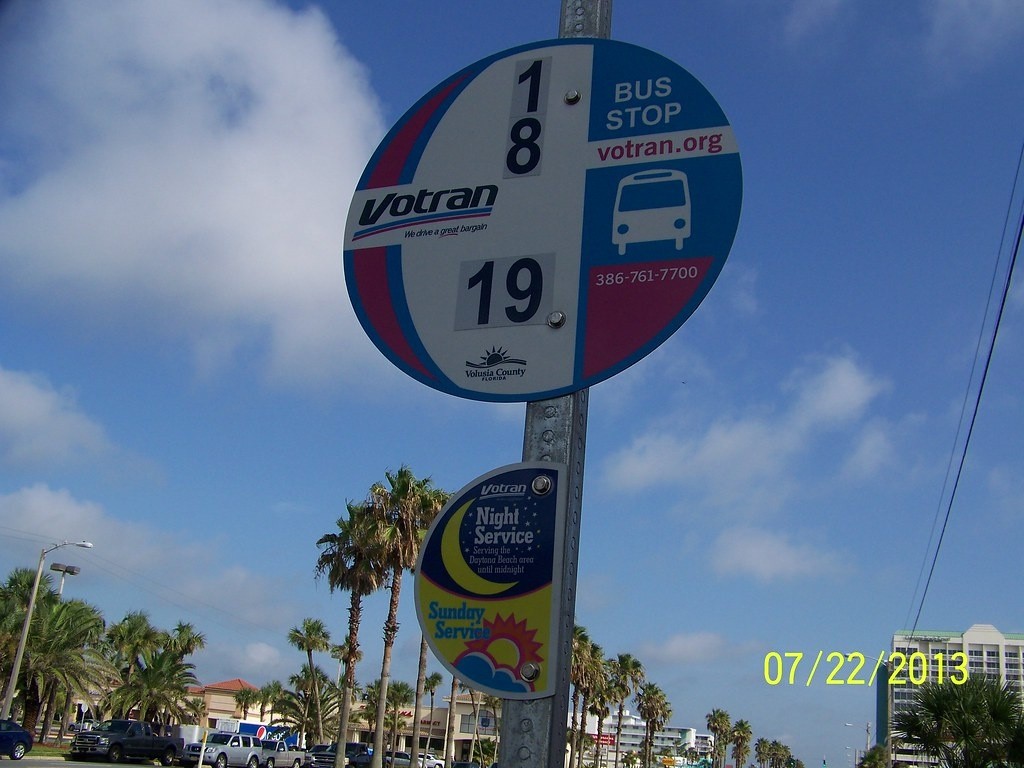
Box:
[845,723,870,751]
[846,747,859,768]
[0,539,93,717]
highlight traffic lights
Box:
[791,762,794,766]
[824,761,825,766]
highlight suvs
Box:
[307,743,372,768]
[184,731,263,768]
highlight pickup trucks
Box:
[69,720,184,765]
[261,739,306,768]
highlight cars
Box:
[385,750,445,768]
[68,719,101,732]
[0,720,32,760]
